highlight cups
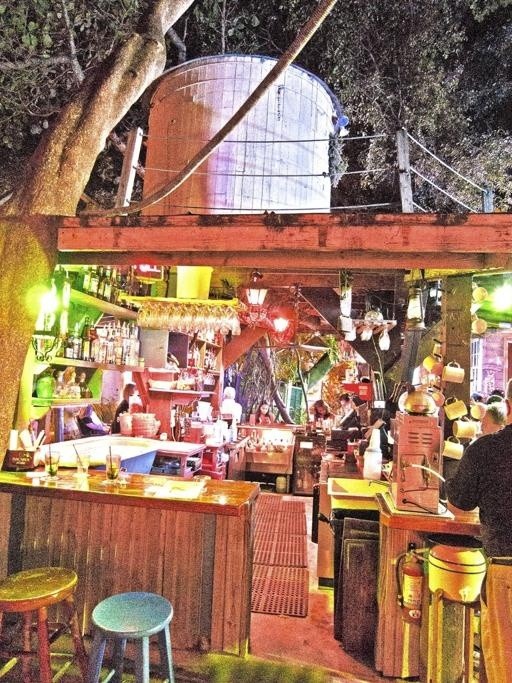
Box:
[76,455,89,476]
[471,282,488,302]
[44,455,59,478]
[105,454,121,481]
[443,396,487,461]
[421,352,465,383]
[471,312,487,334]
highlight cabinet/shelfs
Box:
[139,328,223,441]
[18,263,145,446]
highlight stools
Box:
[0,566,90,683]
[90,592,176,683]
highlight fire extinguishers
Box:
[394,542,424,624]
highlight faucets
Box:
[368,470,392,489]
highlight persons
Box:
[313,393,366,442]
[111,383,136,434]
[77,406,110,436]
[256,400,275,425]
[67,371,87,390]
[444,379,512,683]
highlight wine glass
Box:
[134,302,242,336]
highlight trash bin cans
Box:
[327,477,390,641]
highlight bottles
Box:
[76,265,148,311]
[296,470,316,491]
[54,370,96,398]
[63,316,141,367]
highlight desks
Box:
[153,441,207,476]
[374,491,483,679]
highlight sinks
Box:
[328,477,393,512]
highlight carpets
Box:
[0,620,369,683]
[249,492,310,620]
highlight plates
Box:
[132,413,155,438]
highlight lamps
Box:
[245,269,270,308]
[405,268,428,333]
